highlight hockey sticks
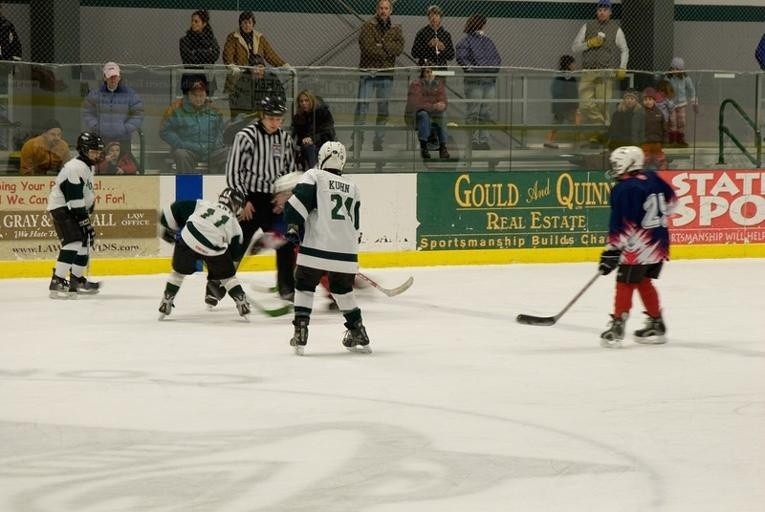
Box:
[88,209,103,294]
[358,272,413,296]
[170,235,293,316]
[517,270,604,326]
[251,284,278,293]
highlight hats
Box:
[103,62,120,79]
[672,57,685,70]
[559,55,575,67]
[642,86,656,100]
[623,88,641,102]
[107,138,120,150]
[189,80,206,91]
[249,54,265,67]
[597,1,611,9]
[46,119,61,131]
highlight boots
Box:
[70,267,98,292]
[49,267,69,292]
[342,307,369,349]
[679,132,689,148]
[634,309,666,337]
[158,283,180,315]
[600,312,629,341]
[669,131,679,147]
[289,307,312,345]
[228,284,250,316]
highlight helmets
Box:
[218,188,243,213]
[610,146,645,174]
[260,95,287,117]
[318,141,347,172]
[78,132,104,165]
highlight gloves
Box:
[693,103,700,114]
[598,260,616,274]
[281,222,303,246]
[283,63,296,75]
[83,226,98,241]
[587,36,604,49]
[163,227,174,246]
[615,68,626,80]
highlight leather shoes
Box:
[439,146,449,158]
[421,148,431,158]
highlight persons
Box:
[276,140,372,354]
[205,94,296,313]
[545,0,698,171]
[21,120,72,177]
[1,16,23,60]
[456,15,501,149]
[160,10,336,175]
[404,63,451,158]
[46,132,107,300]
[160,188,251,322]
[411,5,455,78]
[83,61,144,152]
[97,137,138,174]
[600,144,679,344]
[351,0,404,150]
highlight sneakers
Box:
[481,142,489,150]
[602,128,611,147]
[349,141,362,151]
[591,140,599,149]
[472,142,481,150]
[544,141,559,148]
[373,141,382,150]
[205,280,221,305]
